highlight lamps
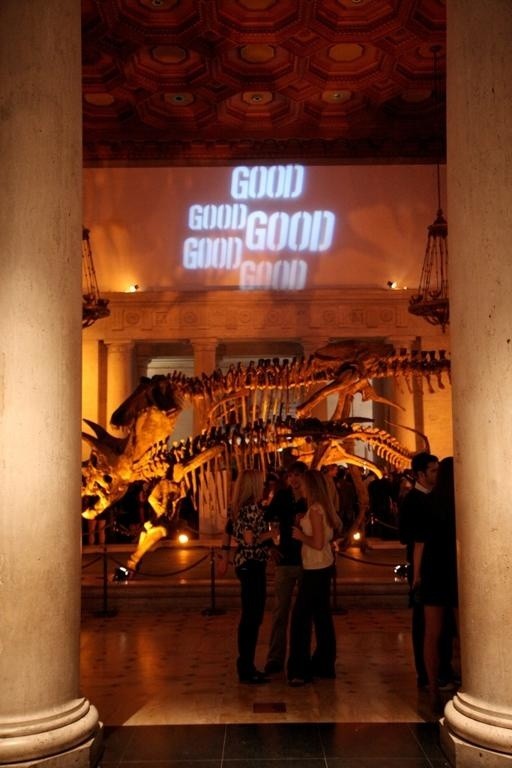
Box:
[113,566,129,581]
[130,285,138,292]
[82,226,111,327]
[408,46,449,334]
[387,281,396,288]
[394,565,408,578]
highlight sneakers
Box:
[237,660,338,687]
[425,673,461,715]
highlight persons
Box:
[86,452,461,713]
